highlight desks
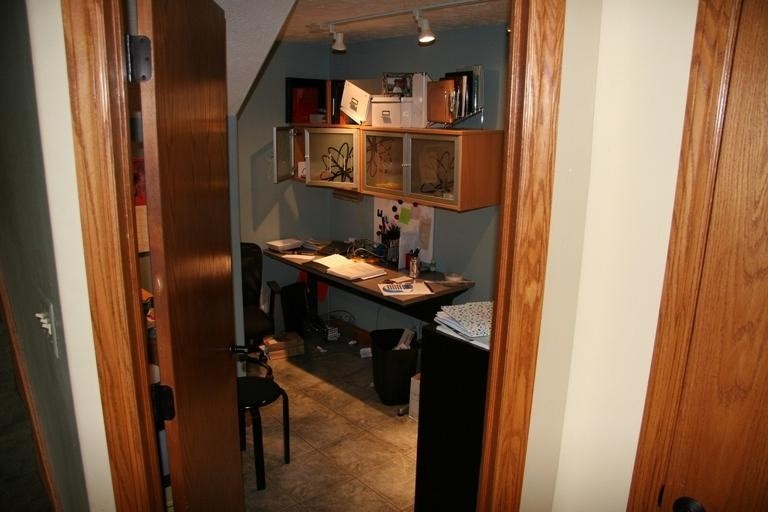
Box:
[263,240,476,417]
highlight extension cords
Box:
[328,327,338,341]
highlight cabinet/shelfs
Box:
[273,123,504,213]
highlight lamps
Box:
[307,0,494,51]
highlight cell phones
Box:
[383,283,413,292]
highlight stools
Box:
[236,354,290,490]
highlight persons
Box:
[393,77,410,95]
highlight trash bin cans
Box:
[369,329,418,407]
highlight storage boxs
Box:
[340,78,381,126]
[371,95,401,127]
[263,331,305,361]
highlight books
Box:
[433,298,497,352]
[425,61,485,125]
[264,238,434,299]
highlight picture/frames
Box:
[381,72,413,96]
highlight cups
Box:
[410,257,420,279]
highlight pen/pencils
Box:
[410,247,424,260]
[424,281,433,292]
